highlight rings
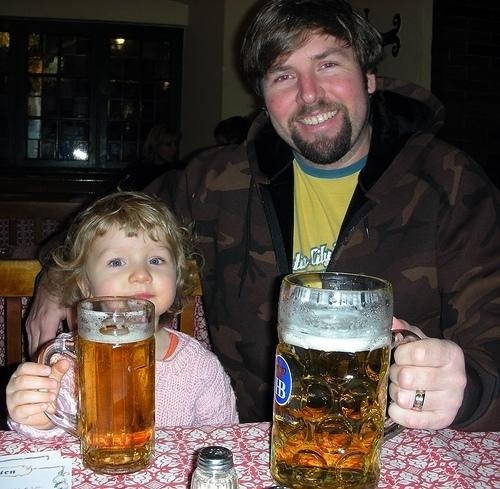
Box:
[411,389,426,413]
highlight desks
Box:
[0,420,499,487]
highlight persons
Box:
[213,115,252,144]
[120,124,186,190]
[22,1,499,434]
[4,189,243,442]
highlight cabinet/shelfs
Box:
[0,18,186,182]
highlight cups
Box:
[37,296,156,475]
[270,271,422,489]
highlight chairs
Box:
[1,260,212,368]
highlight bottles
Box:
[190,445,239,489]
